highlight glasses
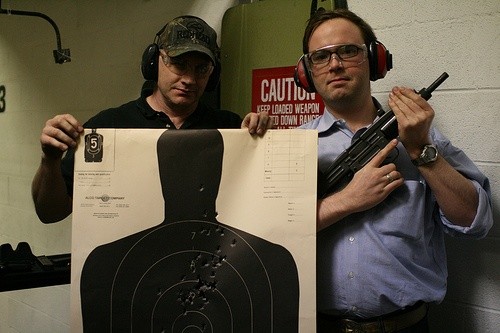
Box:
[305,44,369,76]
[309,45,363,65]
[159,49,215,80]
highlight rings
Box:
[386,173,394,182]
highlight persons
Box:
[31,15,274,225]
[292,9,494,333]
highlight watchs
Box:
[411,144,438,165]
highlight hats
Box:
[156,17,220,68]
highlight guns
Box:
[318,72,449,198]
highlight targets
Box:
[79,128,299,333]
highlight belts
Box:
[318,304,427,333]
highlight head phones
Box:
[141,16,223,93]
[292,9,394,94]
